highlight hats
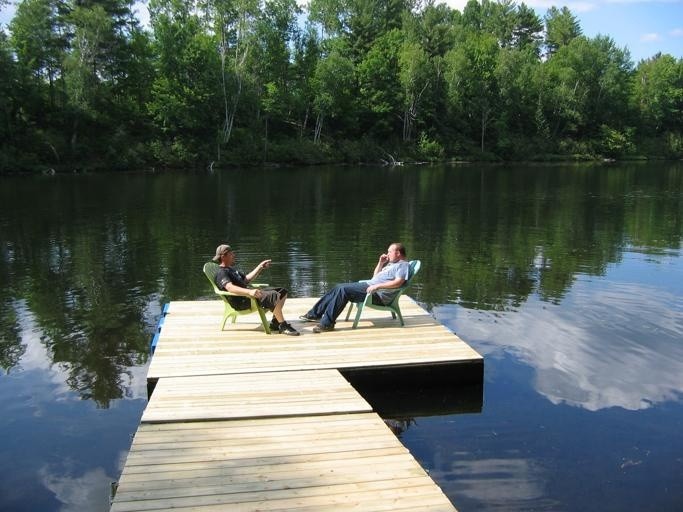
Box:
[212,243,232,261]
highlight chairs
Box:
[199,260,276,336]
[343,258,422,329]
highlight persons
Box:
[212,244,300,336]
[299,242,412,333]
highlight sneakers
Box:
[269,319,300,337]
[300,313,319,322]
[312,323,335,333]
